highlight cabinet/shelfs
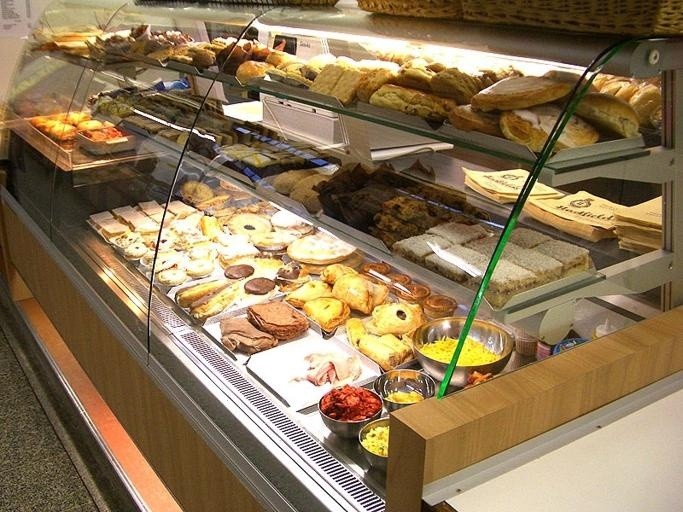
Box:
[0,0,683,512]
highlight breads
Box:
[276,159,437,226]
[148,36,291,86]
[221,262,456,383]
[191,120,342,184]
[90,179,362,322]
[31,111,136,156]
[265,52,663,156]
[30,22,188,63]
[88,76,244,147]
[370,176,591,307]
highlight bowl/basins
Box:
[318,387,383,438]
[358,416,390,472]
[373,369,436,413]
[405,317,515,387]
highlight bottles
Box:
[593,317,616,339]
